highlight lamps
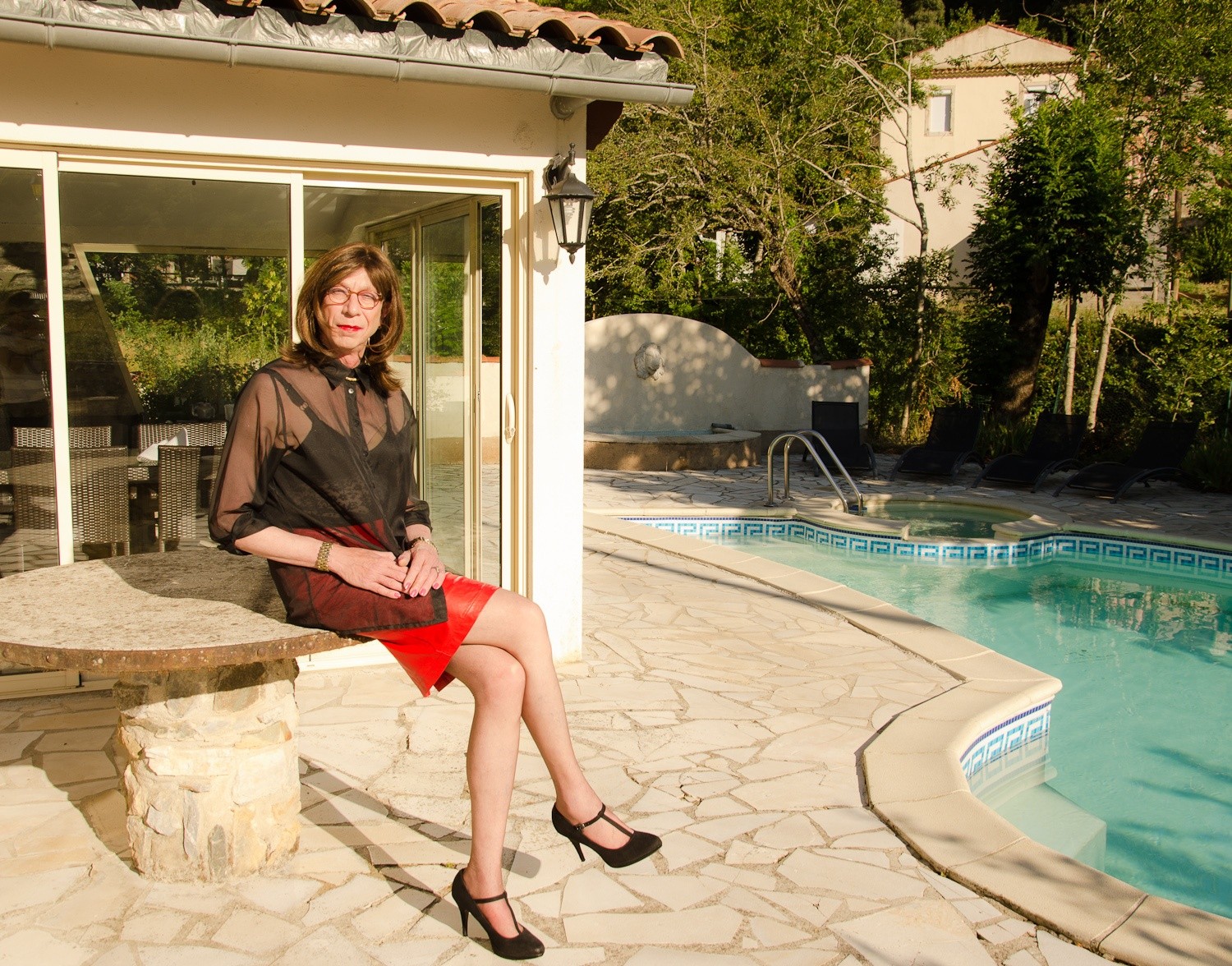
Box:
[544,143,597,264]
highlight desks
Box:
[0,550,375,882]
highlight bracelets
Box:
[316,542,334,572]
[410,536,440,558]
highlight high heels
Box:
[452,869,544,960]
[552,802,662,868]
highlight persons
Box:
[206,242,662,960]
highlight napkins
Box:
[137,428,189,463]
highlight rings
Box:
[431,566,439,576]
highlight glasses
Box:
[326,286,383,311]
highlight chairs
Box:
[12,422,227,573]
